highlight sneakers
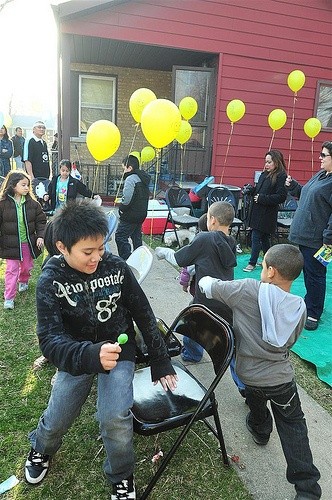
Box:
[110,473,137,500]
[24,447,50,485]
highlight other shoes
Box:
[18,283,28,292]
[3,300,14,309]
[304,316,318,330]
[243,265,254,272]
[255,261,261,266]
[246,411,268,445]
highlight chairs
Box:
[107,303,234,500]
[125,246,153,287]
[277,194,297,239]
[162,185,199,249]
[208,187,243,250]
[103,211,116,251]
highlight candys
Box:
[117,333,128,345]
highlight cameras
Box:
[241,184,252,195]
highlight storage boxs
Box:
[142,210,174,236]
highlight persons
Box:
[155,201,246,399]
[197,243,322,500]
[0,121,152,386]
[243,150,288,273]
[284,141,332,330]
[23,201,179,500]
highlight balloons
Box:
[128,87,157,124]
[286,69,307,93]
[141,98,182,149]
[303,117,322,139]
[141,145,156,162]
[129,150,141,163]
[175,120,193,145]
[85,120,122,162]
[267,108,287,131]
[226,98,246,123]
[4,114,12,128]
[178,96,199,121]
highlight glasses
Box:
[35,126,46,129]
[320,152,332,158]
